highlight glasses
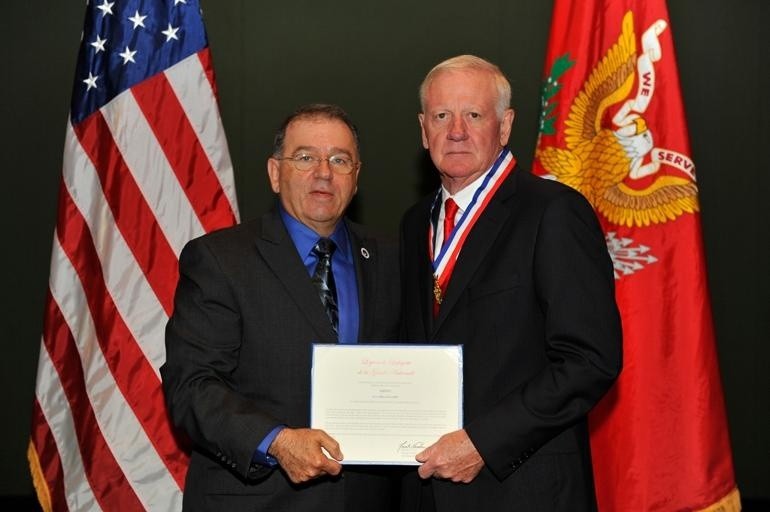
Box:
[275,150,359,176]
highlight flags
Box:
[530,1,747,511]
[26,0,244,512]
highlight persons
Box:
[157,99,414,511]
[400,52,623,511]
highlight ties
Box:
[443,197,460,243]
[312,237,338,339]
